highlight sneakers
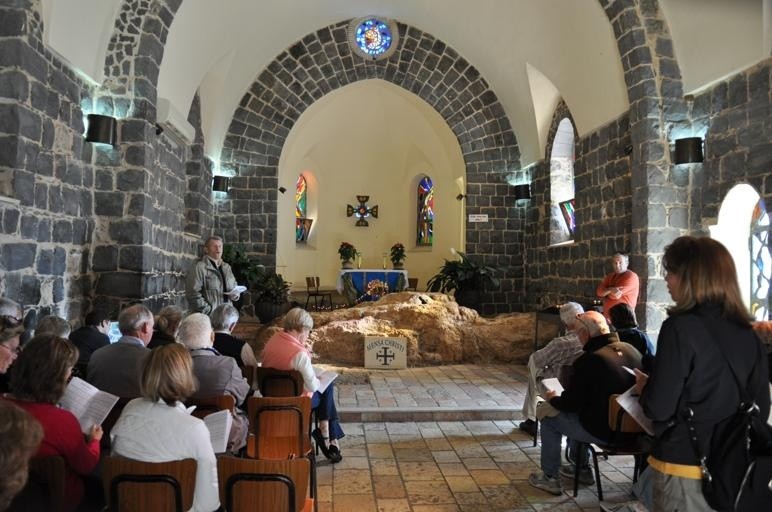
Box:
[600,500,639,512]
[529,472,563,495]
[559,464,596,485]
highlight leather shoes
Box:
[312,428,342,461]
[520,418,536,436]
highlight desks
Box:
[336,269,409,303]
[535,304,603,351]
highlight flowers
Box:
[366,280,388,296]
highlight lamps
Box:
[278,187,286,194]
[674,137,704,165]
[514,184,531,200]
[212,176,229,192]
[456,194,466,201]
[85,113,117,146]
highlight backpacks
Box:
[686,308,772,512]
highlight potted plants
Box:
[389,243,407,269]
[337,242,356,269]
[425,252,508,314]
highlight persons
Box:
[1,297,345,512]
[518,301,771,511]
[600,252,640,326]
[186,236,241,315]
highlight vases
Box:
[371,294,380,301]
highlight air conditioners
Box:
[156,98,196,148]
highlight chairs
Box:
[406,278,418,291]
[534,365,608,461]
[573,394,646,512]
[305,277,333,312]
